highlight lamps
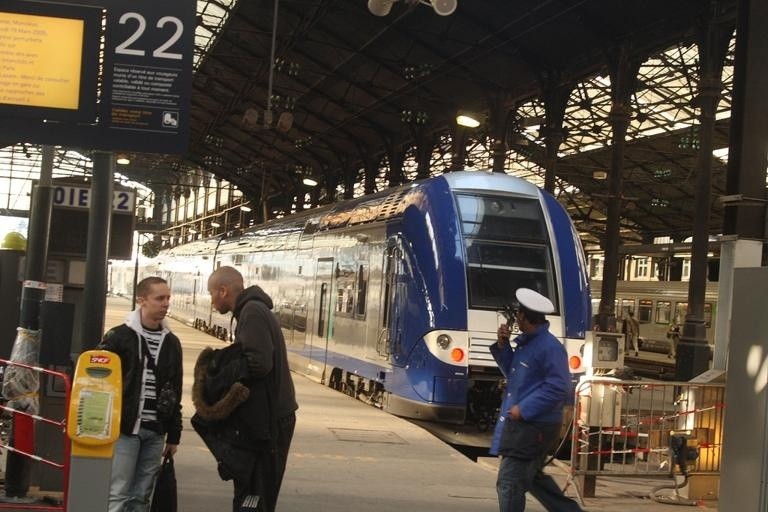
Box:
[242,0,294,134]
[367,0,458,18]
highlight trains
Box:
[138,167,595,435]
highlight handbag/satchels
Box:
[150,450,178,512]
[499,416,548,460]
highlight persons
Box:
[199,265,299,511]
[622,310,640,357]
[666,318,680,359]
[488,287,584,510]
[95,276,185,511]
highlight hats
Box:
[515,287,555,314]
